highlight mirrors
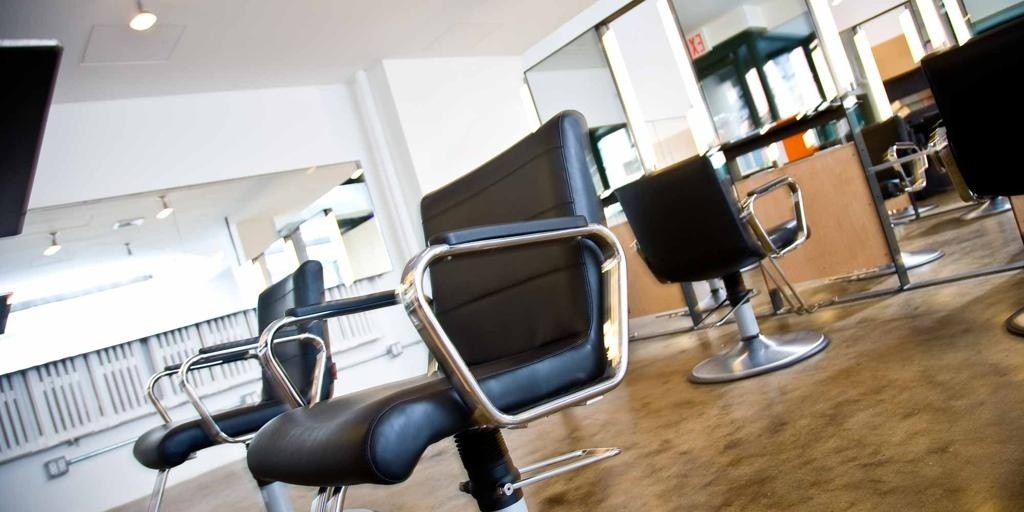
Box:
[523,0,1024,290]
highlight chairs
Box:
[920,14,1024,340]
[257,110,630,512]
[614,154,831,385]
[133,259,336,512]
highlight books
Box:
[721,94,841,150]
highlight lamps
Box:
[155,194,171,221]
[44,231,59,255]
[127,2,156,33]
